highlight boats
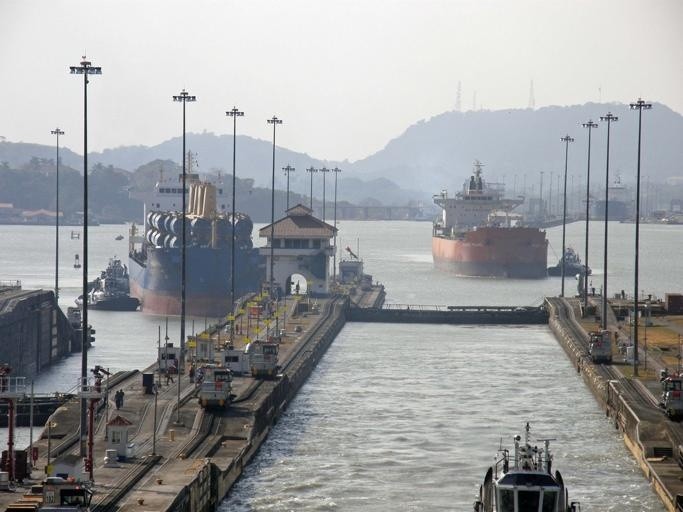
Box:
[475,422,583,511]
[548,248,591,279]
[428,160,548,281]
[71,230,140,311]
[122,150,263,321]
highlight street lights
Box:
[49,128,63,305]
[559,133,575,298]
[169,89,196,372]
[626,98,652,372]
[227,107,244,351]
[265,117,282,300]
[283,164,341,284]
[66,55,102,456]
[580,120,598,313]
[598,113,618,330]
[507,170,575,221]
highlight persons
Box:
[113,390,121,410]
[165,367,174,386]
[188,367,195,383]
[117,389,124,408]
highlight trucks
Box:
[586,331,613,364]
[246,342,278,379]
[661,373,683,417]
[197,364,233,409]
